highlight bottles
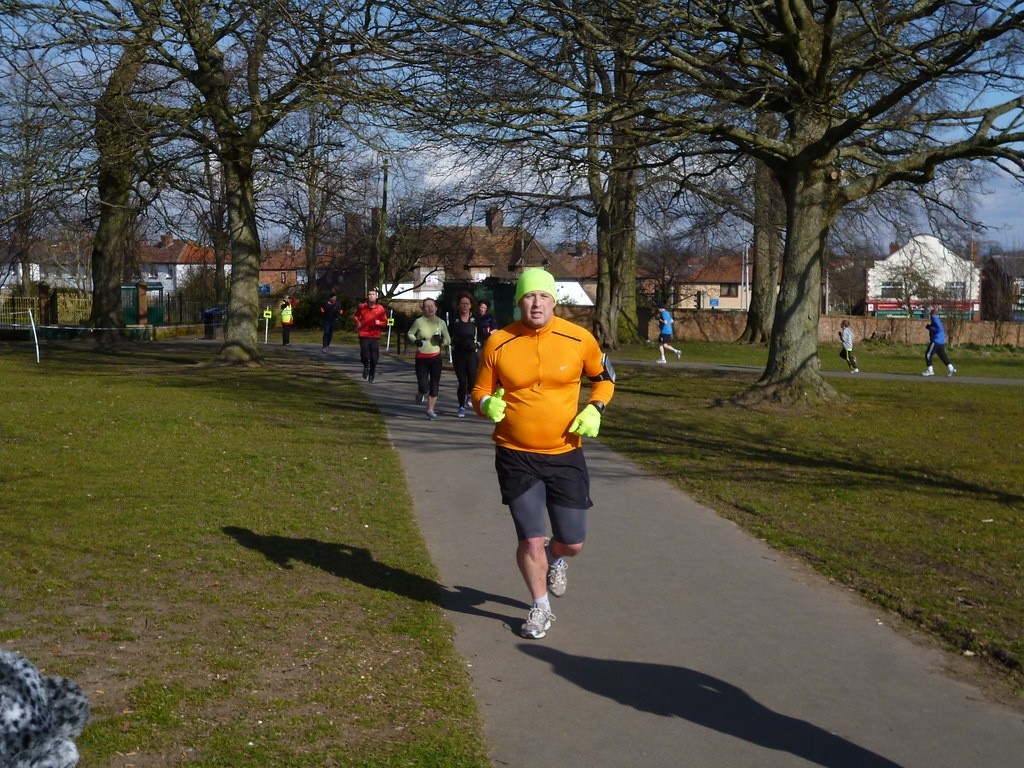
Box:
[418,337,426,351]
[434,328,440,335]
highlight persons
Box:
[474,299,498,370]
[922,309,957,377]
[469,268,617,640]
[279,294,295,346]
[320,292,343,353]
[407,297,452,420]
[655,301,682,363]
[445,291,485,418]
[353,289,388,384]
[839,320,860,374]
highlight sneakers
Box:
[425,410,437,418]
[369,370,375,383]
[520,602,556,638]
[466,394,474,410]
[416,391,424,404]
[362,368,369,379]
[544,537,569,597]
[457,406,466,417]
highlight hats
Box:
[515,267,557,306]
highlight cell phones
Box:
[602,352,616,383]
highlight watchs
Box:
[590,399,606,415]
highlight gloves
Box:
[415,337,425,347]
[430,333,442,346]
[467,342,475,351]
[568,403,602,437]
[482,387,507,423]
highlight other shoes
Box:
[656,359,667,364]
[322,346,328,353]
[849,367,859,373]
[676,349,682,360]
[922,369,934,376]
[947,369,957,376]
